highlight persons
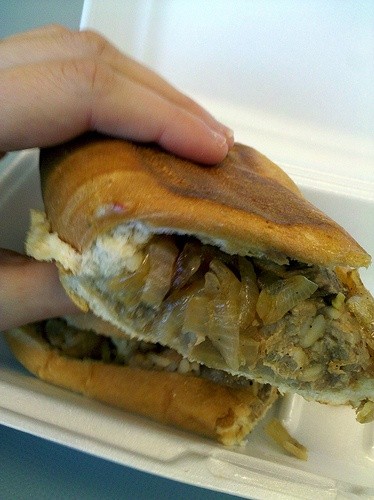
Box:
[0,22,236,329]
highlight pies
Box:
[7,121,372,443]
